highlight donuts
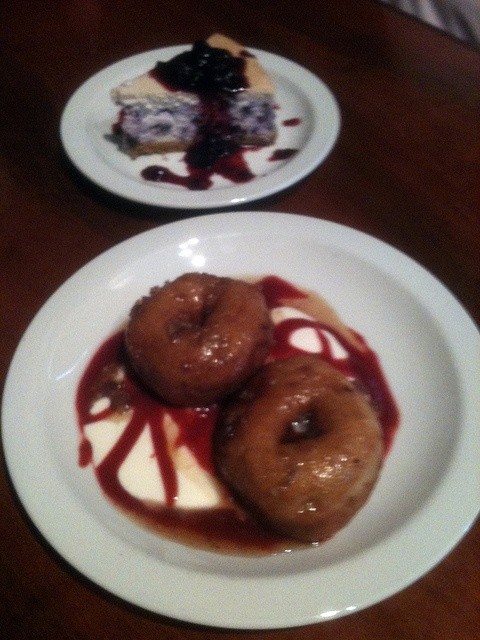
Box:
[212,358,384,542]
[125,275,270,407]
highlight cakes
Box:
[110,30,279,155]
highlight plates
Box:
[59,44,341,209]
[0,209,480,628]
